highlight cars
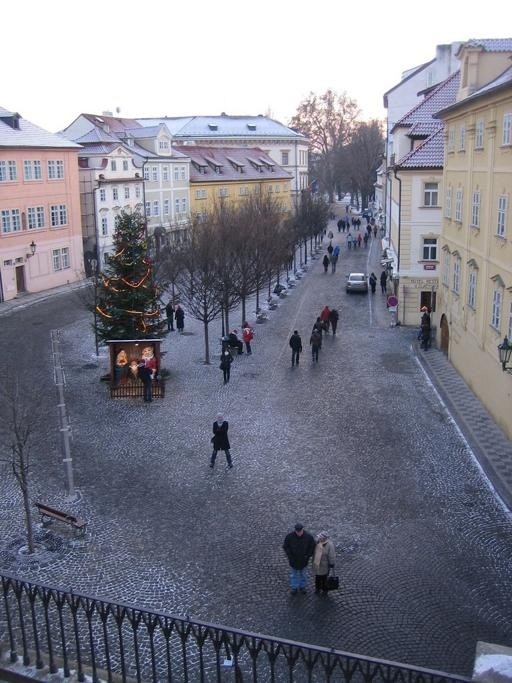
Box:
[344,272,370,293]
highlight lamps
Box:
[496,333,512,373]
[30,240,37,255]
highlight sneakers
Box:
[290,586,328,597]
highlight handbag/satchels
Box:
[323,575,340,591]
[218,363,225,370]
[210,435,217,443]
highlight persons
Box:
[309,306,340,363]
[208,413,234,468]
[381,271,388,294]
[321,205,378,273]
[114,347,131,385]
[137,344,157,380]
[229,330,244,355]
[288,330,302,367]
[166,299,176,331]
[136,359,153,402]
[220,347,234,384]
[175,306,184,332]
[370,272,377,294]
[242,321,253,355]
[421,321,430,352]
[312,531,337,596]
[282,523,316,595]
[417,308,432,340]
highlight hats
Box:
[294,523,304,531]
[317,531,330,539]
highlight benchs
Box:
[37,502,88,538]
